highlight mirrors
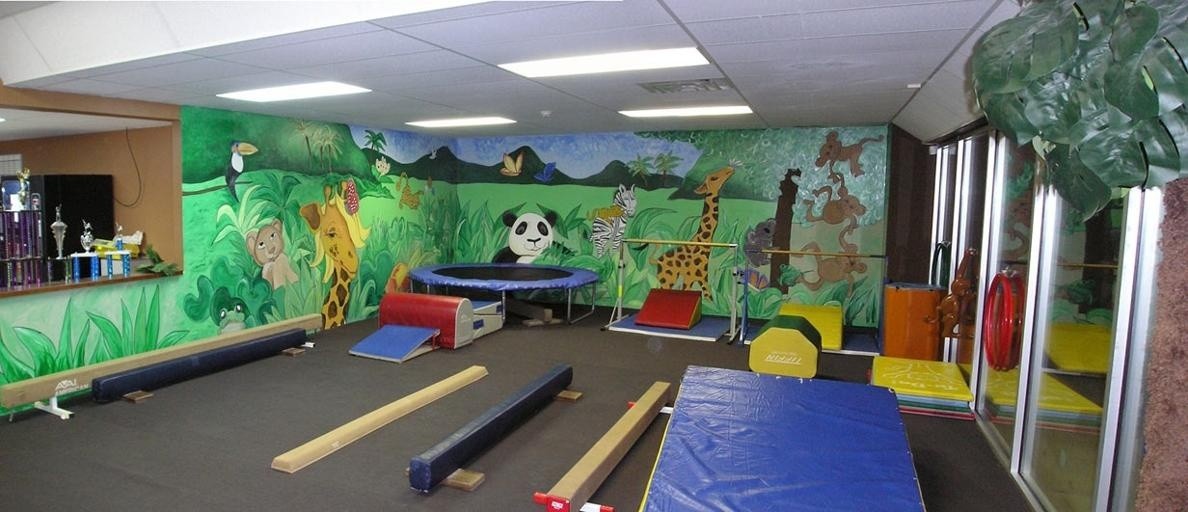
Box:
[928,110,1159,512]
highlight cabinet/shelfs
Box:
[100,257,155,276]
[0,175,114,284]
